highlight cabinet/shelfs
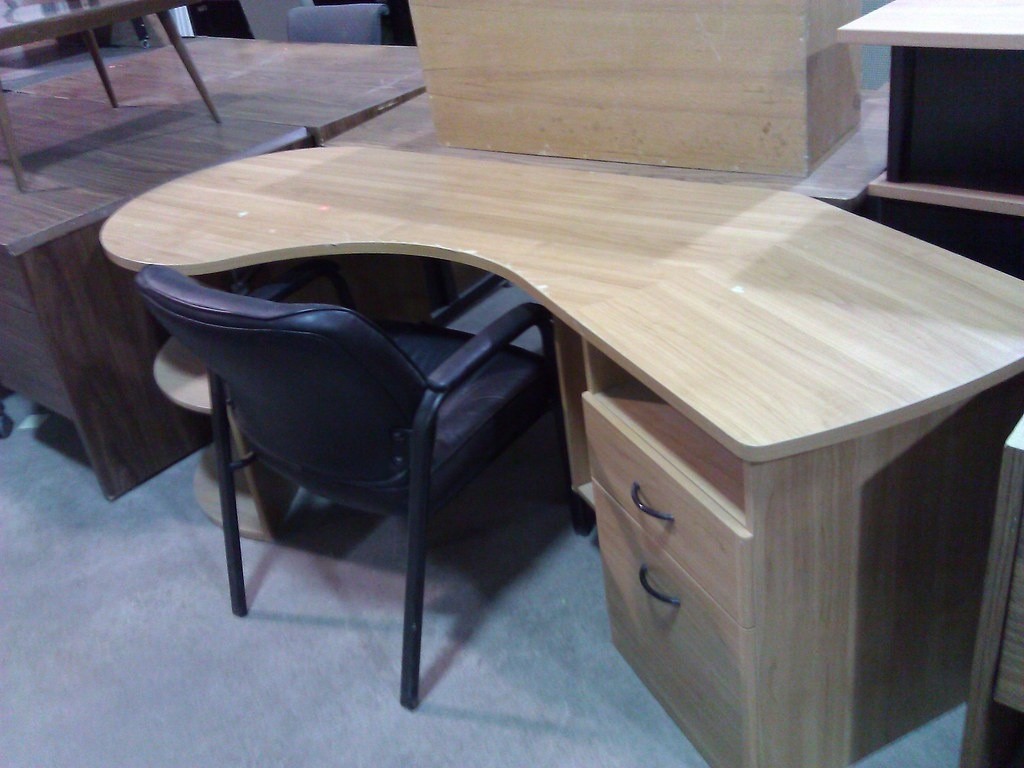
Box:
[407,0,863,179]
[838,1,1024,282]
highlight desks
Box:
[99,145,1024,766]
[319,89,890,225]
[0,0,222,194]
[0,90,313,500]
[15,38,424,141]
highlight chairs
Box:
[133,264,596,711]
[288,3,390,46]
